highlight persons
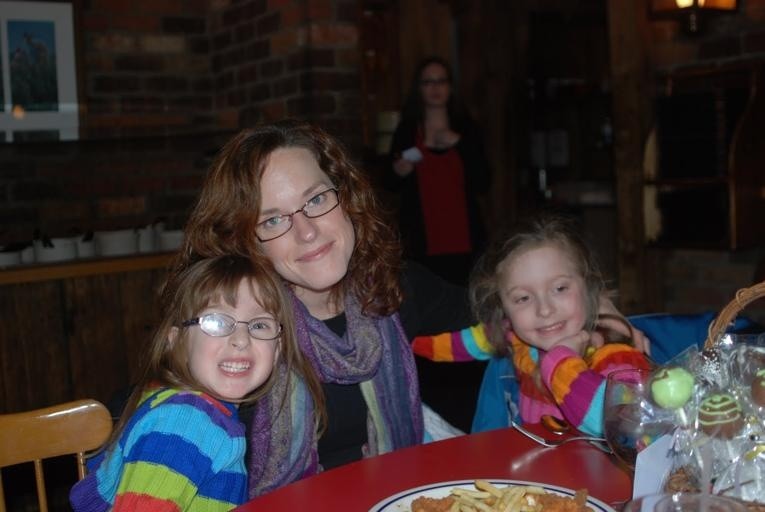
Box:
[410,202,649,453]
[385,51,495,292]
[153,109,651,505]
[67,253,328,511]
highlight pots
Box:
[0,222,186,268]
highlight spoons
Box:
[542,412,613,454]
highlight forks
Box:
[509,419,612,446]
[508,447,555,473]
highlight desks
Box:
[226,421,635,512]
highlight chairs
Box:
[0,400,113,512]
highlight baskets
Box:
[604,277,765,511]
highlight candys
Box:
[649,349,765,494]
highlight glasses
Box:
[179,310,284,341]
[252,188,343,243]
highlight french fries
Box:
[449,479,547,512]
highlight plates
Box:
[366,477,617,511]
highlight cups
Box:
[600,367,695,476]
